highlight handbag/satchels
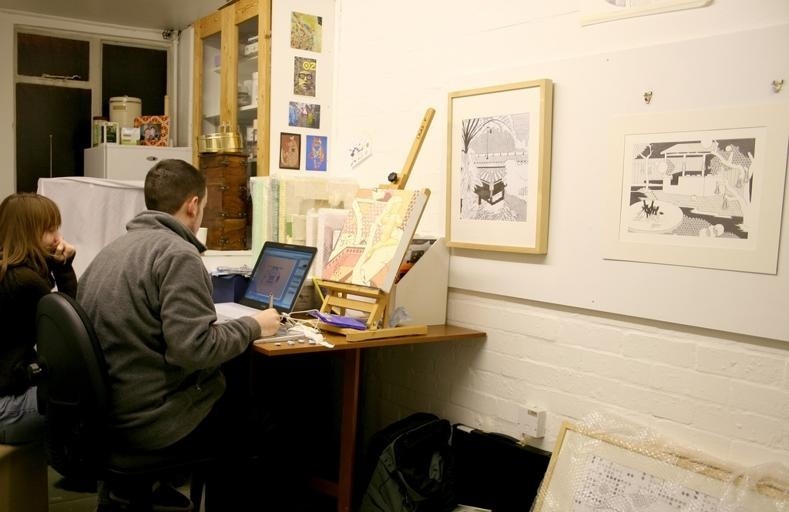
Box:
[452,422,551,512]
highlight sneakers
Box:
[109,486,193,510]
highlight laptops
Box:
[212,240,318,326]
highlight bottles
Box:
[92,116,107,147]
[238,71,258,107]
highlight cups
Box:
[195,227,209,257]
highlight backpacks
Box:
[360,413,451,512]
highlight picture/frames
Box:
[533,421,789,512]
[133,115,170,146]
[602,105,789,276]
[280,132,301,170]
[445,80,553,255]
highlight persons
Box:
[141,126,159,140]
[1,193,194,512]
[76,159,281,512]
[298,69,312,90]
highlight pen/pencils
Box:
[269,291,274,308]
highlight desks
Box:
[250,324,486,511]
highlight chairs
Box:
[39,295,209,512]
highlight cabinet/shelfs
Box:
[193,1,271,176]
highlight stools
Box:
[0,443,48,512]
